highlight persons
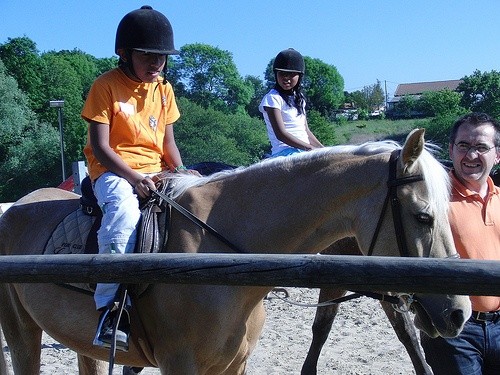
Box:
[420,113,500,375]
[82,5,203,352]
[258,47,325,158]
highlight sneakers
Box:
[98,310,130,348]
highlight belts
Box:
[469,309,500,324]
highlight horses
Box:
[0,127,472,375]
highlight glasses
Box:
[453,141,496,156]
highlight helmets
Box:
[115,5,181,56]
[273,48,305,76]
